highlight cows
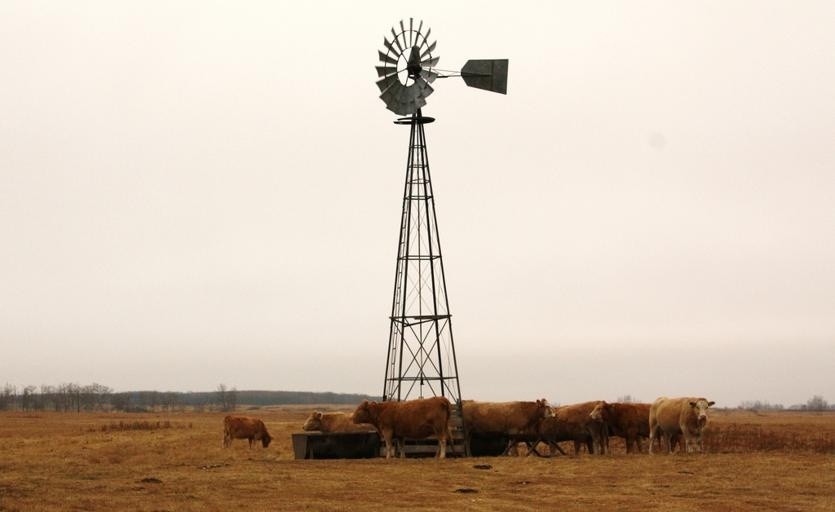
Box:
[301,410,375,433]
[351,396,452,461]
[650,397,716,454]
[459,397,650,458]
[221,414,274,450]
